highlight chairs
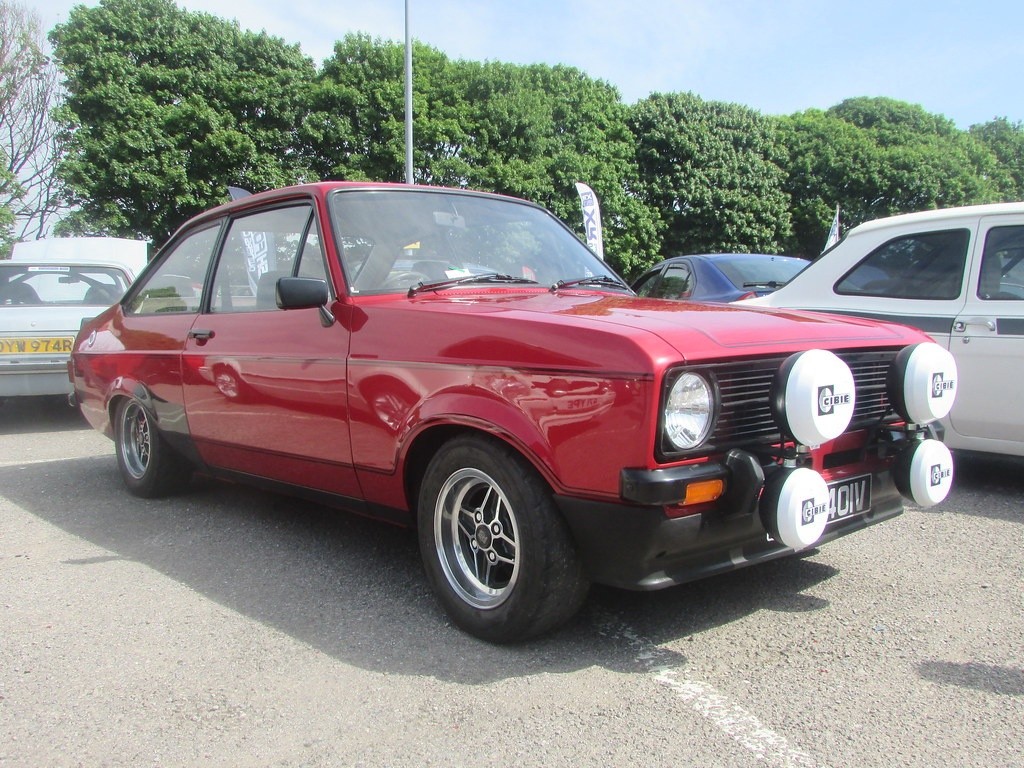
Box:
[82,284,119,305]
[0,283,41,304]
[255,271,296,311]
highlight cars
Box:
[64,181,957,646]
[630,255,867,301]
[0,237,148,395]
[725,196,1024,458]
